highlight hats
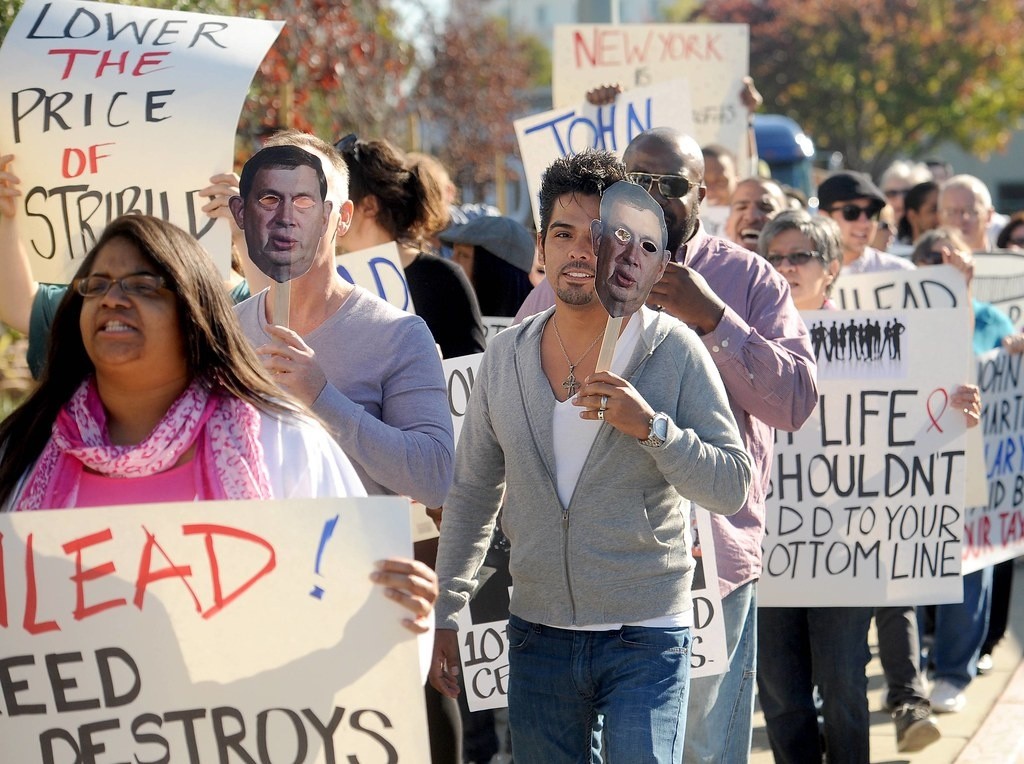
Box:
[439,216,535,272]
[817,172,886,209]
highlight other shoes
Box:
[976,655,991,675]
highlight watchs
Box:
[639,414,668,447]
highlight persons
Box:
[0,154,271,382]
[426,152,751,764]
[230,129,548,763]
[0,214,441,689]
[511,76,1024,764]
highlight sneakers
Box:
[892,703,940,752]
[932,680,964,711]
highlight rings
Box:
[598,411,604,420]
[599,395,608,411]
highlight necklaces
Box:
[553,312,606,401]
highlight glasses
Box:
[75,275,170,297]
[766,251,822,266]
[334,134,365,175]
[918,252,942,264]
[627,171,698,200]
[831,205,881,220]
[883,190,907,199]
